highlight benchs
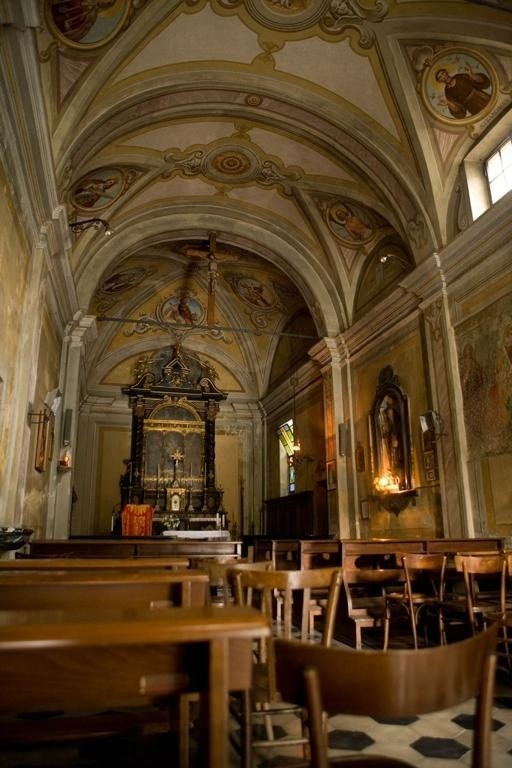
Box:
[0,560,269,768]
[243,538,505,649]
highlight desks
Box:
[30,538,243,560]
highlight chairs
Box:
[441,556,511,664]
[184,558,274,737]
[274,622,498,767]
[384,553,445,650]
[227,566,344,767]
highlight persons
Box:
[75,176,118,207]
[336,209,372,241]
[52,0,116,42]
[435,61,491,119]
